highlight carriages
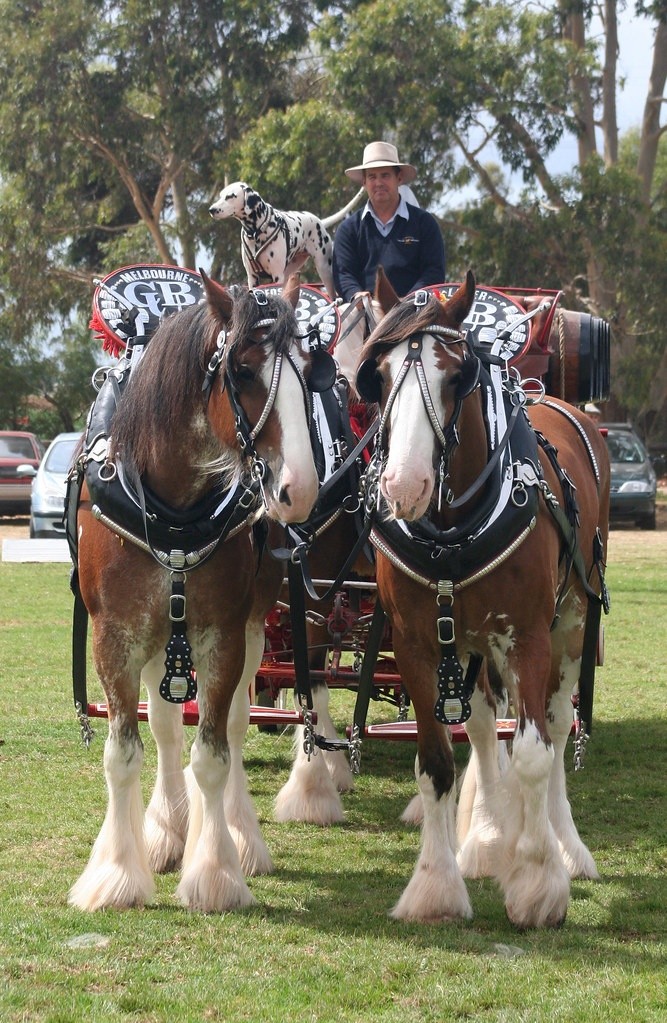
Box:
[52,258,602,932]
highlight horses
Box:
[68,264,612,932]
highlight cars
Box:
[14,432,88,539]
[594,420,656,531]
[0,430,47,518]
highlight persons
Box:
[332,141,443,310]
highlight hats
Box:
[344,142,417,187]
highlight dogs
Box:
[208,180,339,304]
